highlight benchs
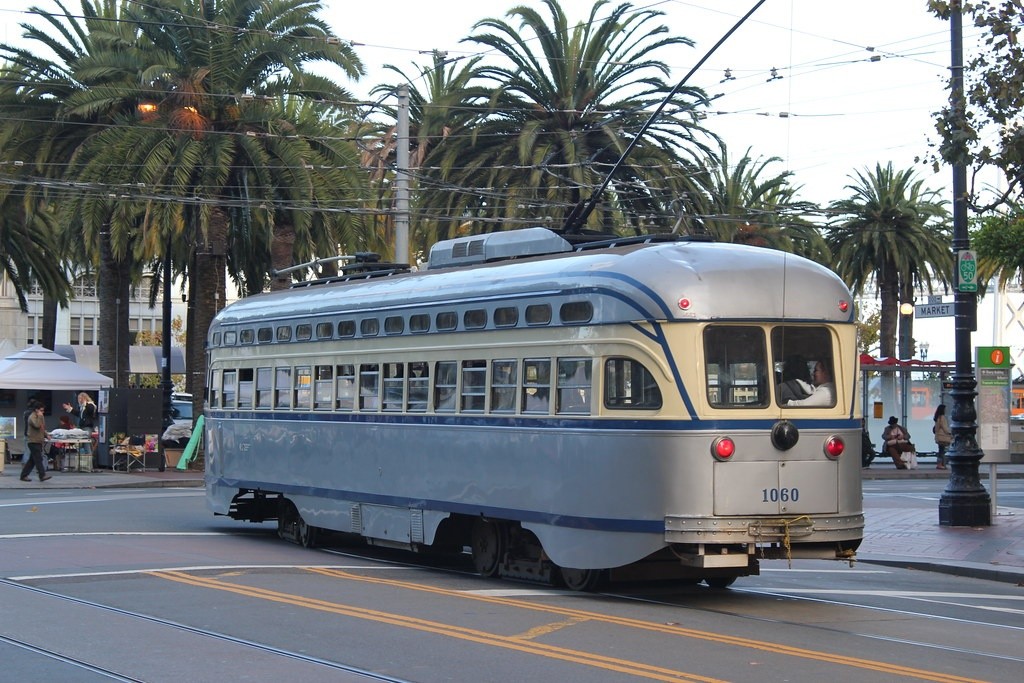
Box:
[867,451,939,457]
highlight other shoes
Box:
[40,475,51,481]
[863,466,871,470]
[897,465,907,470]
[20,478,32,481]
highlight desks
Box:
[43,438,101,473]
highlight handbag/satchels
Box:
[897,442,914,452]
[932,426,936,434]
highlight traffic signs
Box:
[915,303,956,318]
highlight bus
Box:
[209,224,865,586]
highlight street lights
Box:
[901,303,914,431]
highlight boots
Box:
[936,453,948,469]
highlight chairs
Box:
[109,432,146,473]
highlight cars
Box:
[172,399,193,423]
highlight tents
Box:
[0,345,114,464]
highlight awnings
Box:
[46,345,188,373]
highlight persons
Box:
[777,354,816,407]
[862,414,875,470]
[933,405,952,470]
[787,356,837,408]
[882,416,915,470]
[63,392,97,454]
[130,382,151,389]
[20,399,52,482]
[48,415,74,462]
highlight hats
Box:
[888,416,898,424]
